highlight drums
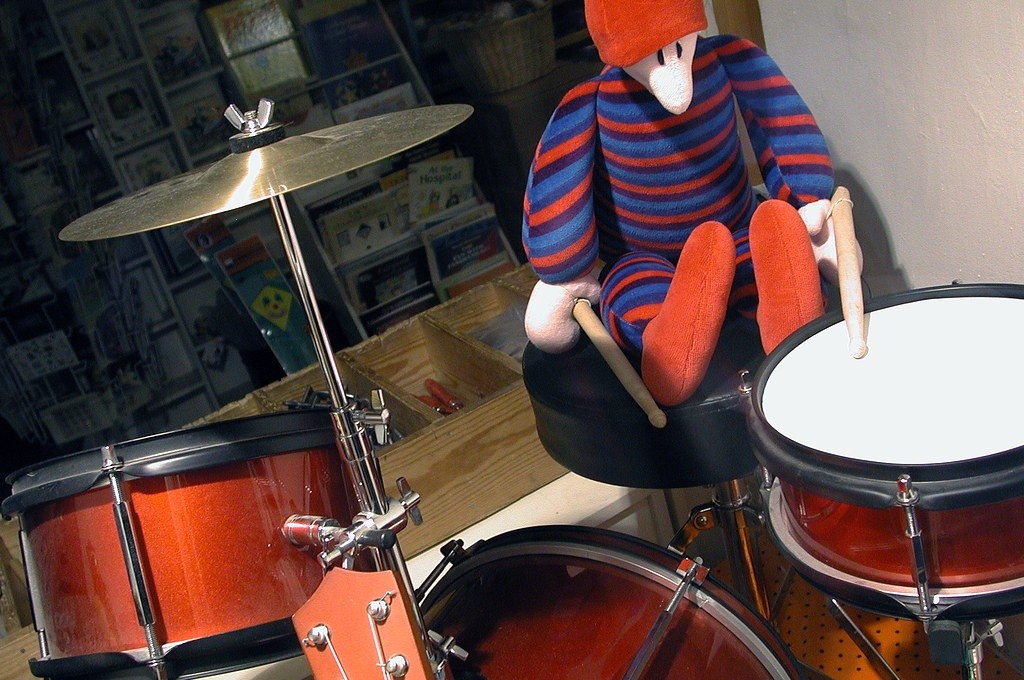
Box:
[410,523,804,680]
[0,407,364,680]
[736,279,1023,622]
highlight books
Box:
[0,0,511,457]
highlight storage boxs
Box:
[441,65,606,235]
[0,260,606,680]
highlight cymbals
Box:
[54,95,481,245]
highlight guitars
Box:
[287,551,438,680]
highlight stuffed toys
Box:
[522,0,864,407]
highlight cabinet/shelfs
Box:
[201,0,523,340]
[45,0,361,411]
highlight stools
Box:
[522,276,876,621]
